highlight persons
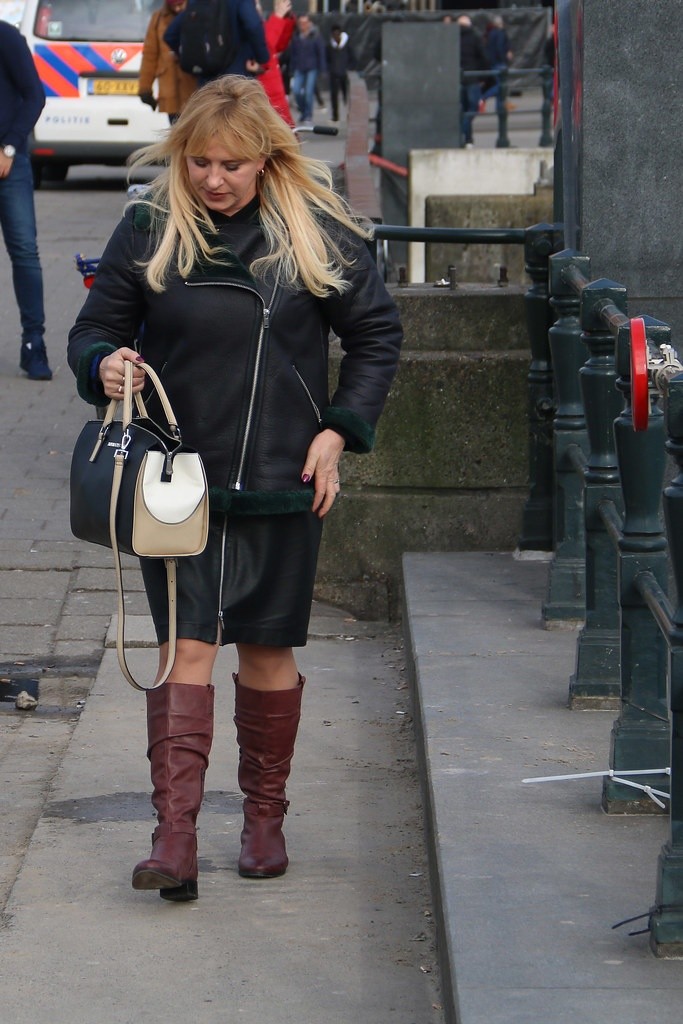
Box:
[0,19,54,387]
[133,0,559,153]
[59,68,409,903]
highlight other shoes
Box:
[330,116,339,124]
[298,115,312,125]
[463,136,475,149]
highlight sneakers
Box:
[19,345,52,380]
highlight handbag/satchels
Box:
[67,349,231,556]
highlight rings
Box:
[333,479,340,483]
[117,376,125,394]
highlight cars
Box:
[15,2,181,186]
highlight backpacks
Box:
[179,1,236,76]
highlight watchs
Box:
[2,144,17,157]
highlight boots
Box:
[233,660,306,879]
[130,685,216,901]
